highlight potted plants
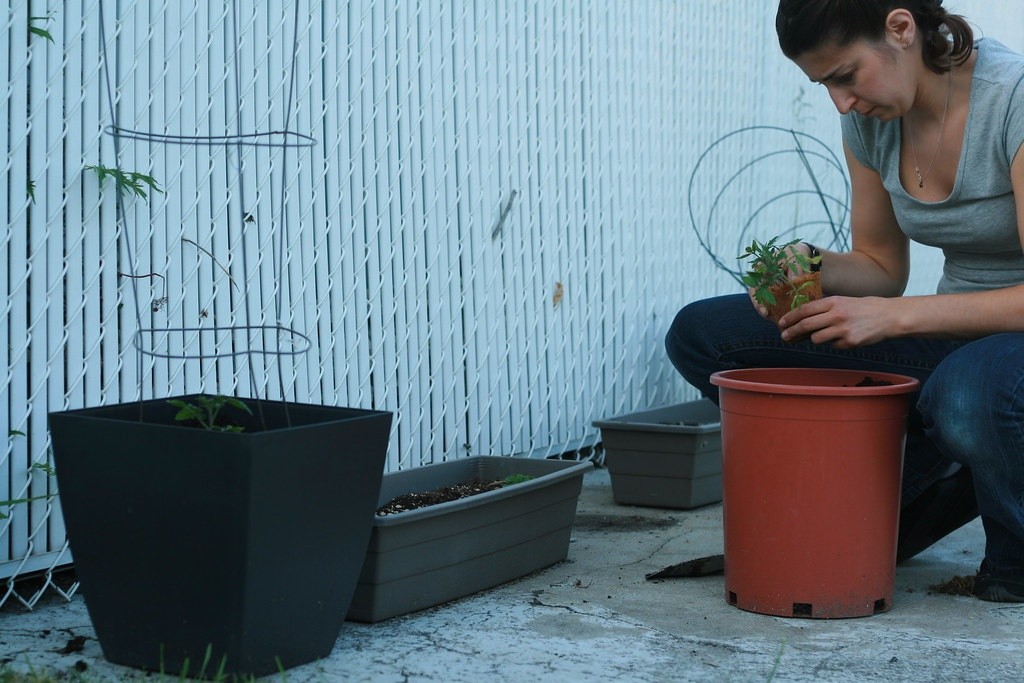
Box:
[592,397,722,510]
[740,236,823,329]
[47,393,394,683]
[346,455,592,623]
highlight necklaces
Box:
[908,65,951,187]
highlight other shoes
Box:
[896,465,981,566]
[973,556,1024,602]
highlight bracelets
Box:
[801,241,823,275]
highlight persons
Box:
[665,0,1024,603]
